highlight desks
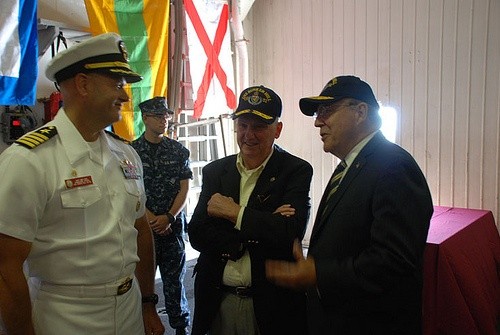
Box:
[423,206,500,335]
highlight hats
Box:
[299,76,380,117]
[44,32,144,83]
[138,96,175,116]
[232,85,283,124]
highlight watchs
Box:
[167,213,175,223]
[142,293,158,304]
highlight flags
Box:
[183,0,239,118]
[84,0,171,141]
[0,0,38,105]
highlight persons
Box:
[0,33,165,335]
[189,87,313,335]
[132,97,191,335]
[267,76,433,335]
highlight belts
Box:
[40,272,135,298]
[222,285,252,299]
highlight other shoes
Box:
[175,328,186,335]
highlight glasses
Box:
[314,101,357,117]
[146,114,171,122]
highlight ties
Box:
[319,159,347,215]
